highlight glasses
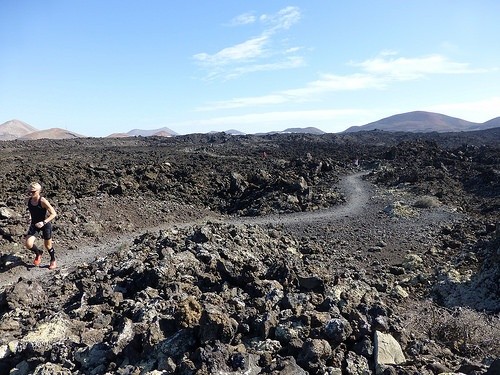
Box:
[27,190,37,194]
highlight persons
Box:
[24,182,57,270]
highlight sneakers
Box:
[49,258,57,269]
[33,251,44,264]
